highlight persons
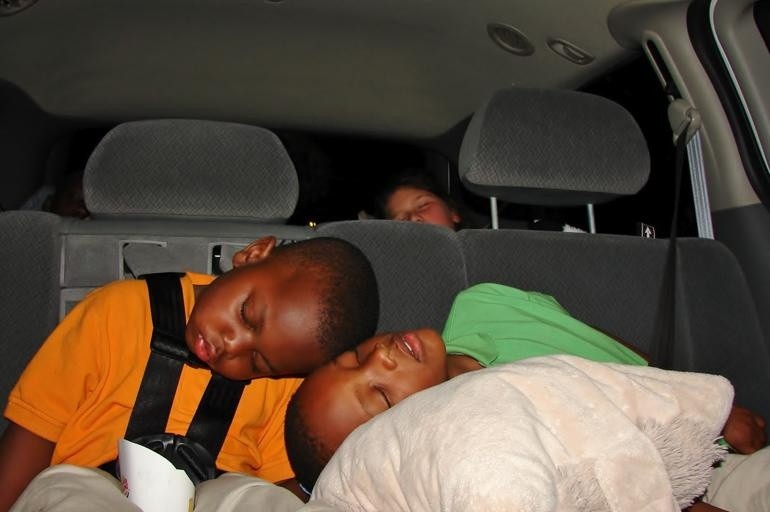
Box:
[0,233,381,512]
[282,279,769,512]
[381,181,462,231]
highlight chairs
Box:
[381,85,770,418]
[1,117,379,453]
